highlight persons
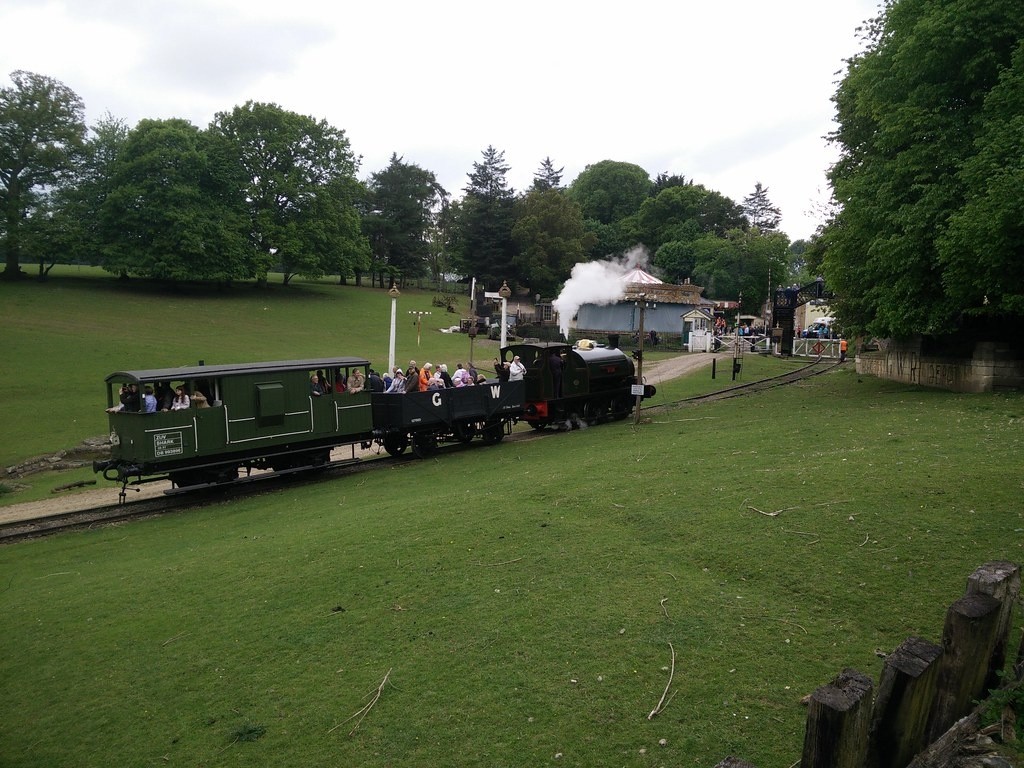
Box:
[494,358,511,382]
[310,359,486,395]
[713,317,750,349]
[549,351,564,398]
[796,325,838,339]
[651,328,656,347]
[106,382,213,412]
[509,356,526,381]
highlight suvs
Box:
[487,313,519,340]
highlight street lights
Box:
[499,281,512,365]
[388,283,400,379]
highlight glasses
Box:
[397,372,401,374]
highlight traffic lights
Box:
[632,349,641,360]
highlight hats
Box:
[513,355,521,360]
[409,365,413,369]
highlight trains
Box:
[91,340,639,495]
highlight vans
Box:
[802,317,842,338]
